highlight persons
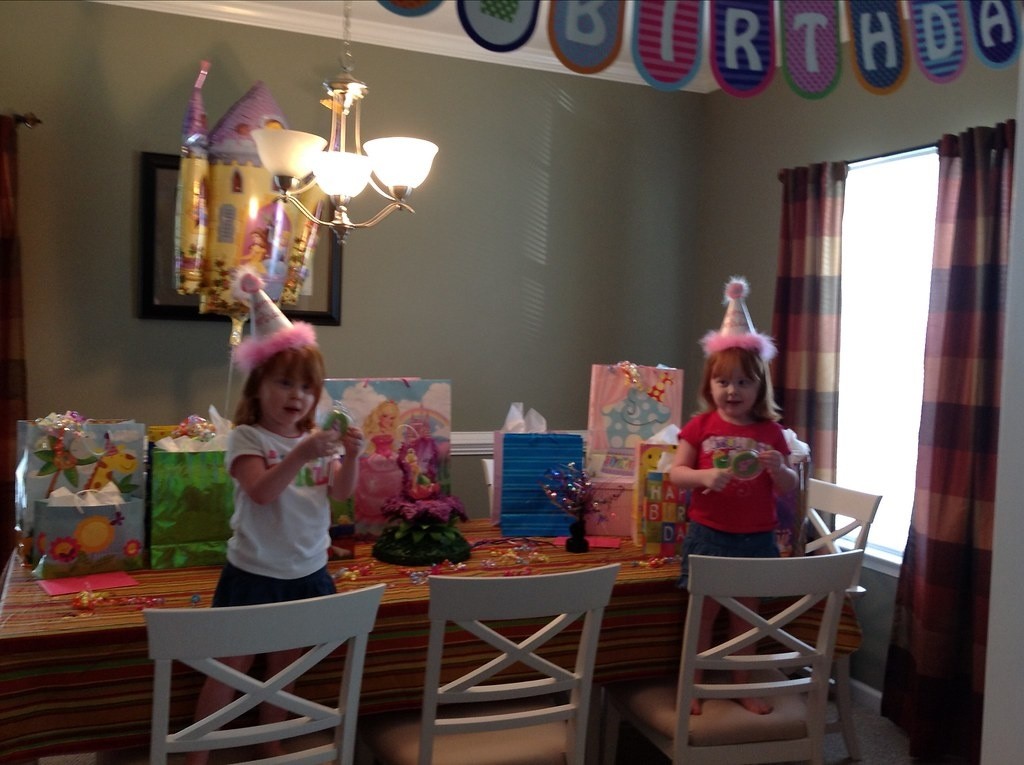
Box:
[668,347,800,716]
[186,346,363,765]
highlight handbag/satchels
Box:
[313,377,452,528]
[588,364,684,455]
[30,489,145,579]
[16,417,146,564]
[147,423,234,569]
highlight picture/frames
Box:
[138,150,342,325]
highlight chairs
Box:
[140,580,387,764]
[598,547,863,764]
[801,477,882,759]
[361,562,622,764]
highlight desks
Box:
[0,515,863,764]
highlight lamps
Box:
[250,0,440,244]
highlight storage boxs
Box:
[148,443,236,569]
[588,476,637,535]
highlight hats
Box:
[230,268,316,374]
[701,278,777,360]
